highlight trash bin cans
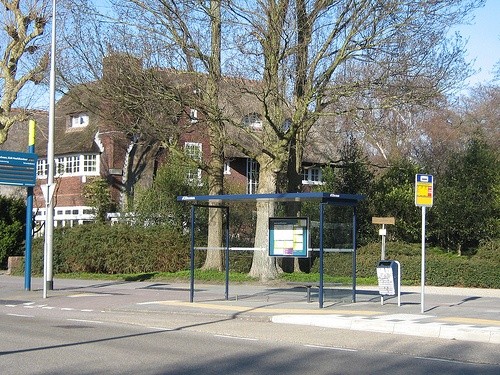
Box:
[376,259,399,296]
